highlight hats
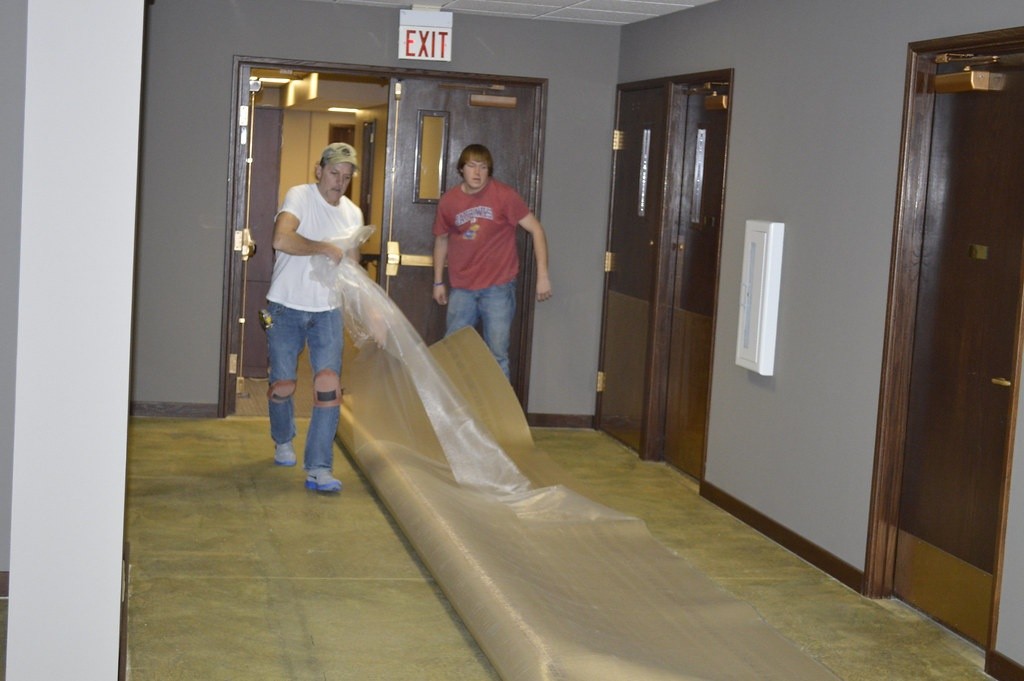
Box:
[323,142,358,169]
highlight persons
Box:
[433,143,553,381]
[265,142,365,491]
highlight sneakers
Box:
[305,468,342,491]
[274,441,297,466]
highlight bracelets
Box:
[433,282,444,286]
